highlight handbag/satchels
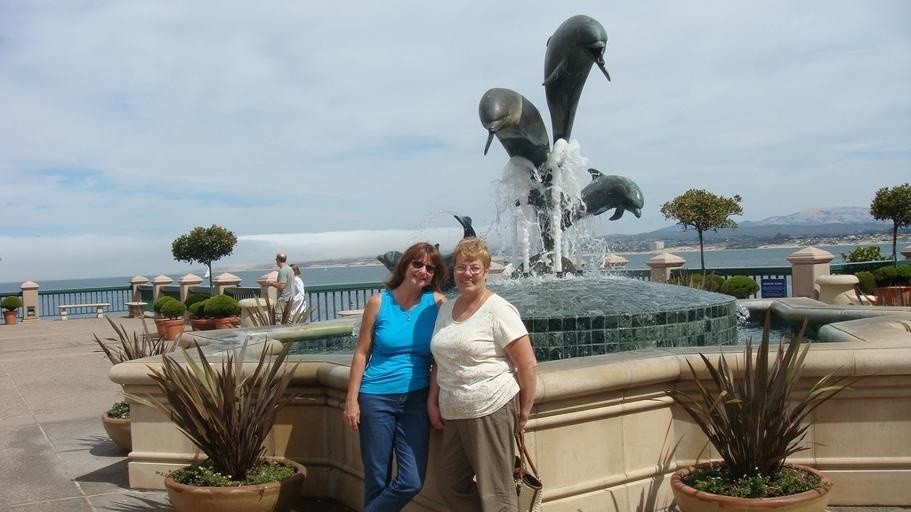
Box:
[507,428,542,512]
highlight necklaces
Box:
[453,289,488,321]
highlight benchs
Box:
[338,309,365,317]
[56,303,111,321]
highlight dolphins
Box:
[541,15,611,222]
[376,215,476,292]
[549,165,645,236]
[479,87,584,282]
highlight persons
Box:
[265,252,296,327]
[342,240,450,511]
[289,262,308,324]
[422,237,540,511]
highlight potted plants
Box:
[89,315,183,452]
[664,304,864,512]
[187,293,243,331]
[871,264,911,307]
[119,336,306,511]
[0,295,21,325]
[149,298,186,341]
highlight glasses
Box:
[452,261,483,276]
[411,260,436,273]
